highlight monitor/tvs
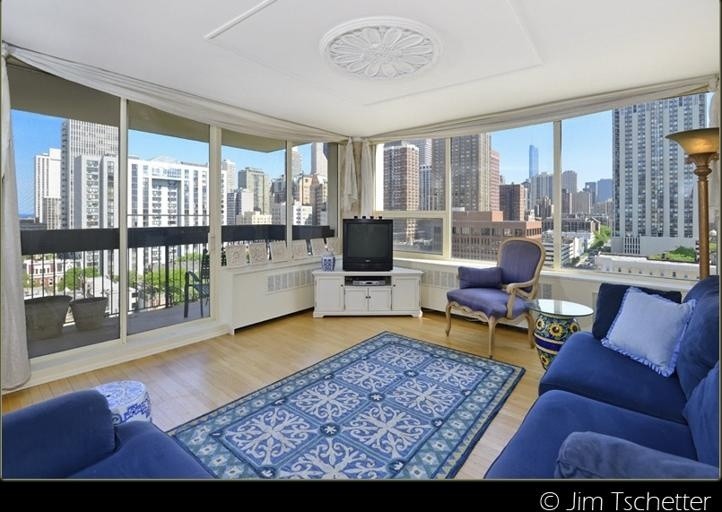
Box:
[342,218,393,271]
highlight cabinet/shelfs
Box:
[312,266,423,317]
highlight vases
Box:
[23,295,72,341]
[69,297,108,329]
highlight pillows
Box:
[458,265,503,289]
[593,283,696,377]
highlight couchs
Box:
[482,268,721,480]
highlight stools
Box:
[93,381,153,426]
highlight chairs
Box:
[183,247,227,318]
[0,391,217,479]
[445,237,546,359]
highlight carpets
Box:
[166,329,526,482]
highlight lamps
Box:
[664,127,720,279]
